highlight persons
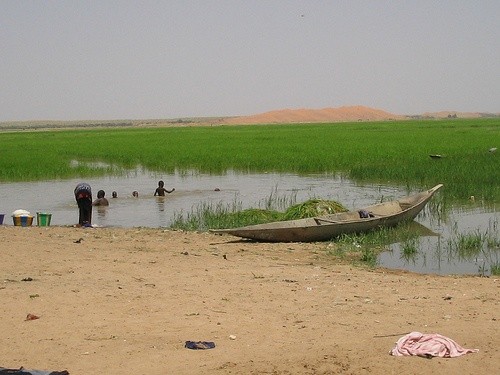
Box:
[112,191,118,198]
[154,181,176,197]
[92,190,109,206]
[215,188,220,191]
[74,183,92,227]
[133,190,139,197]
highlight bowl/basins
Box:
[11,215,35,226]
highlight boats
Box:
[208,183,444,243]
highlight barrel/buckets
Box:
[36,212,52,226]
[0,213,5,225]
